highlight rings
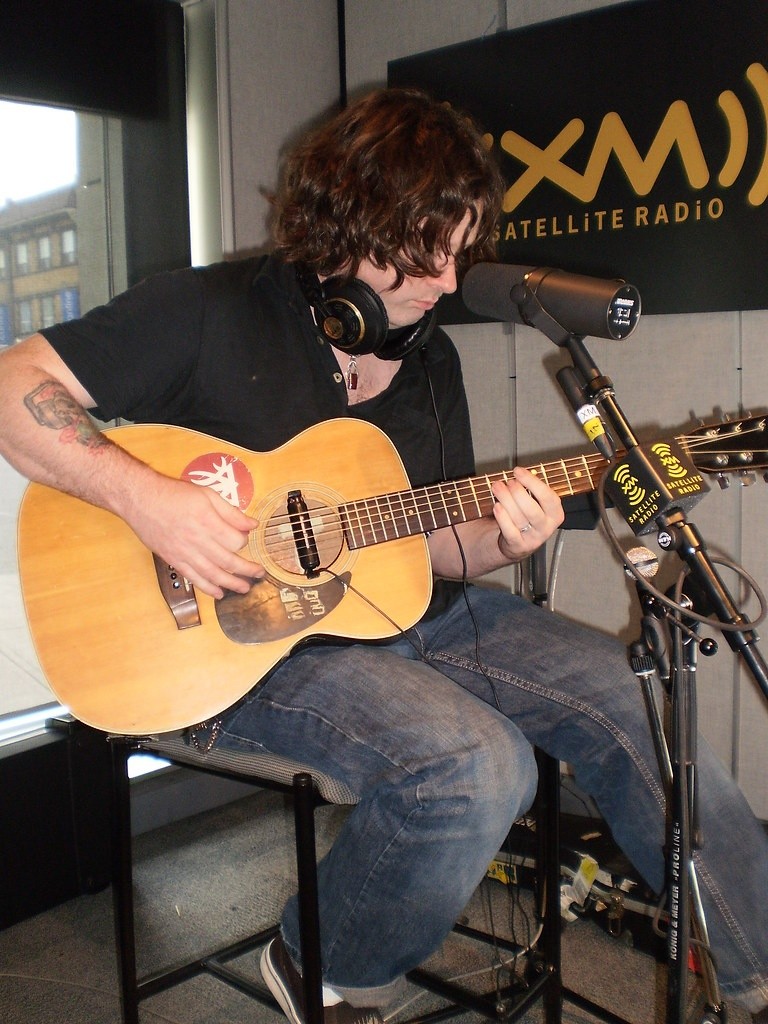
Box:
[519,522,533,534]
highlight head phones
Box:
[293,256,437,361]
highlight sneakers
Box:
[258,936,386,1024]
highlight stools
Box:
[104,730,565,1024]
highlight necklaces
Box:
[341,355,367,393]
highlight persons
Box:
[0,88,767,1024]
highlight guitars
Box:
[15,410,768,738]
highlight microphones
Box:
[624,547,672,685]
[463,262,642,340]
